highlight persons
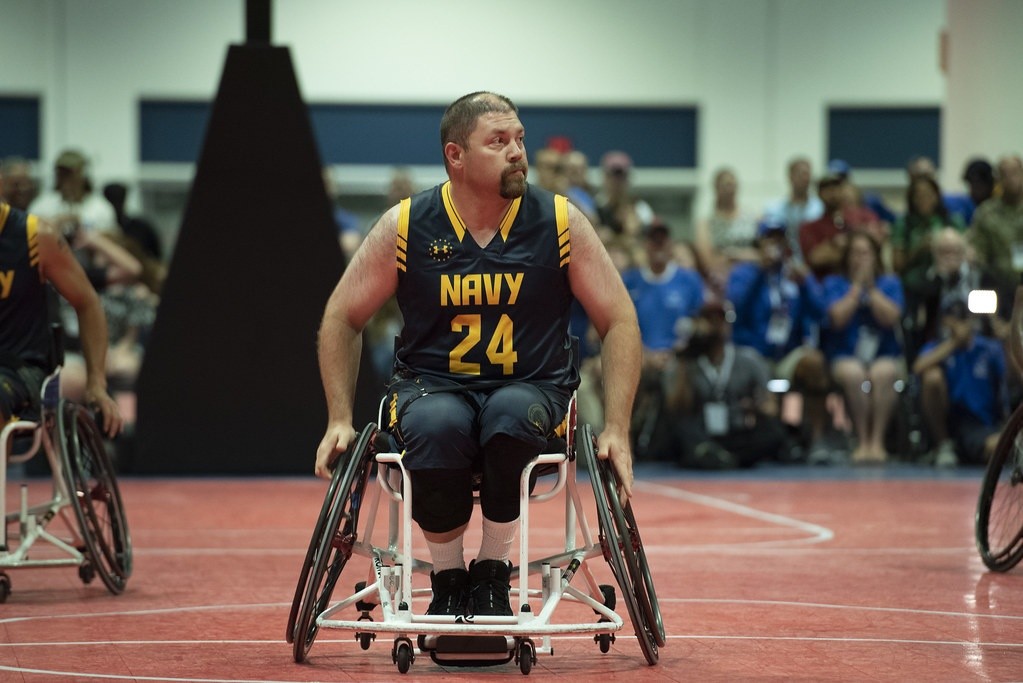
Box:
[316,92,641,616]
[322,169,414,379]
[0,150,165,436]
[0,202,123,440]
[531,143,1023,467]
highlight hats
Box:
[549,137,571,153]
[643,218,670,242]
[759,218,788,240]
[604,152,631,173]
[945,295,967,316]
[817,173,840,190]
[961,159,992,185]
[827,159,848,177]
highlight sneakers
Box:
[425,568,467,619]
[468,558,513,617]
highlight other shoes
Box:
[681,426,957,469]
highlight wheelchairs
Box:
[0,323,134,604]
[285,384,668,676]
[975,402,1023,574]
